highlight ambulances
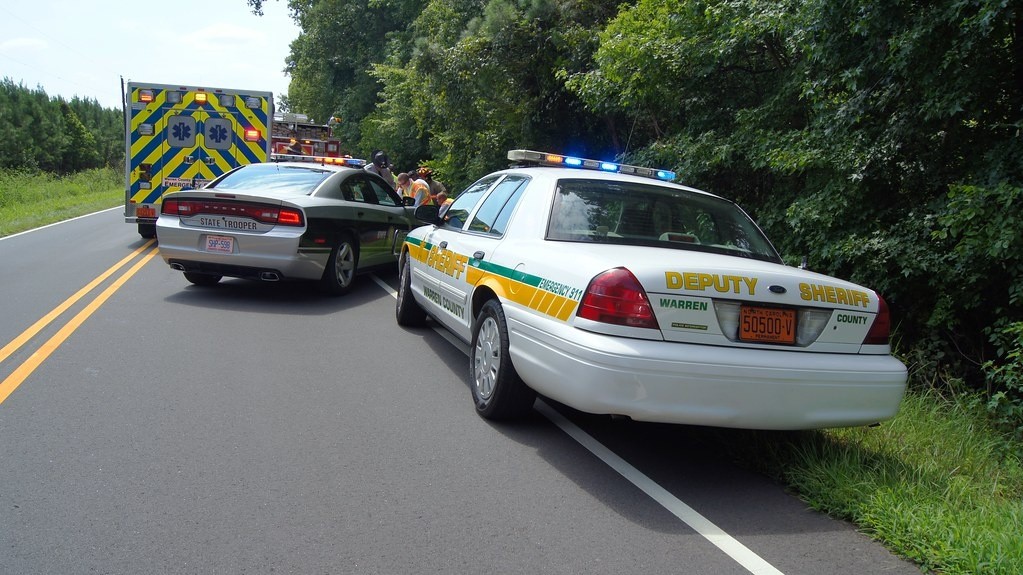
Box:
[118,76,275,238]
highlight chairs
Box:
[614,209,656,234]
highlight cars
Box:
[397,148,909,432]
[156,156,431,295]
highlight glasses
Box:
[398,181,407,185]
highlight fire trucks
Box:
[268,112,367,172]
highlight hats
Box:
[371,150,390,168]
[283,141,305,155]
[389,163,394,166]
[416,168,433,178]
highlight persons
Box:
[397,167,448,206]
[436,191,454,217]
[363,149,396,201]
[387,164,399,192]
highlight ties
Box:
[378,171,383,177]
